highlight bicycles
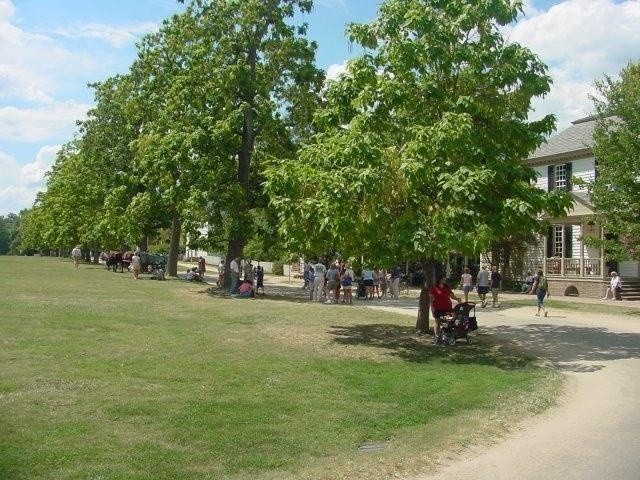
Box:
[390,278,410,296]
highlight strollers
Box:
[437,301,479,346]
[356,278,368,300]
[308,272,327,301]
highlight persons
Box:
[475,265,489,307]
[199,256,206,276]
[429,274,460,339]
[302,258,399,304]
[229,257,265,297]
[603,271,622,300]
[216,260,225,285]
[72,245,81,270]
[521,270,549,317]
[186,269,202,282]
[460,268,473,304]
[490,266,502,308]
[151,268,165,280]
[100,253,107,263]
[131,252,140,279]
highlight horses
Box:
[122,251,134,271]
[105,251,124,273]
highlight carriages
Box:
[102,250,168,274]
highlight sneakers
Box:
[481,300,502,308]
[602,297,616,300]
[534,311,548,317]
